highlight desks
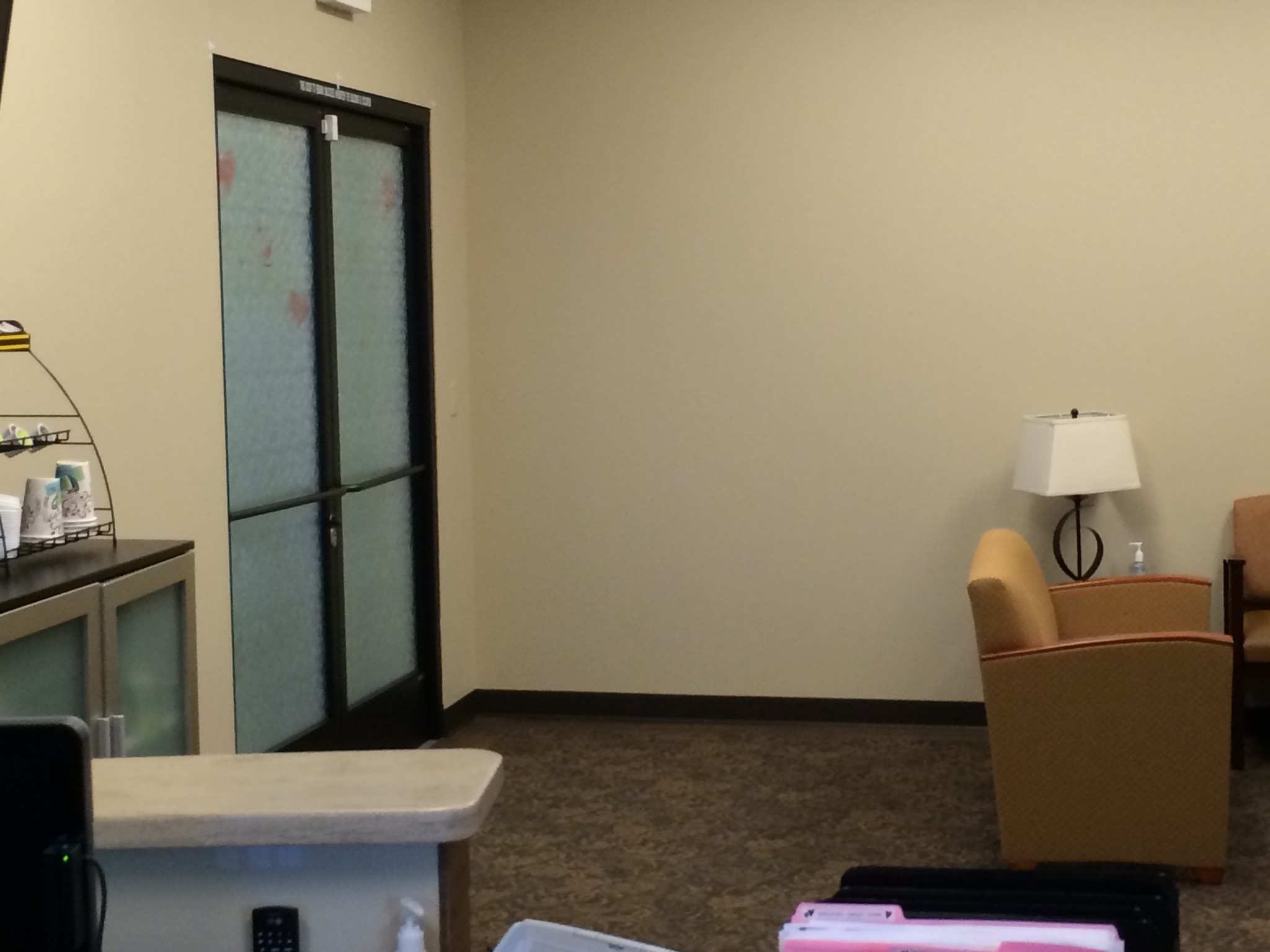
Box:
[93,748,503,952]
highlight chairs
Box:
[967,528,1234,883]
[1223,494,1270,772]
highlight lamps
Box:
[1013,409,1141,580]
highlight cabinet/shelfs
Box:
[0,320,117,575]
[0,537,200,760]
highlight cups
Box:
[0,460,101,562]
[0,424,54,457]
